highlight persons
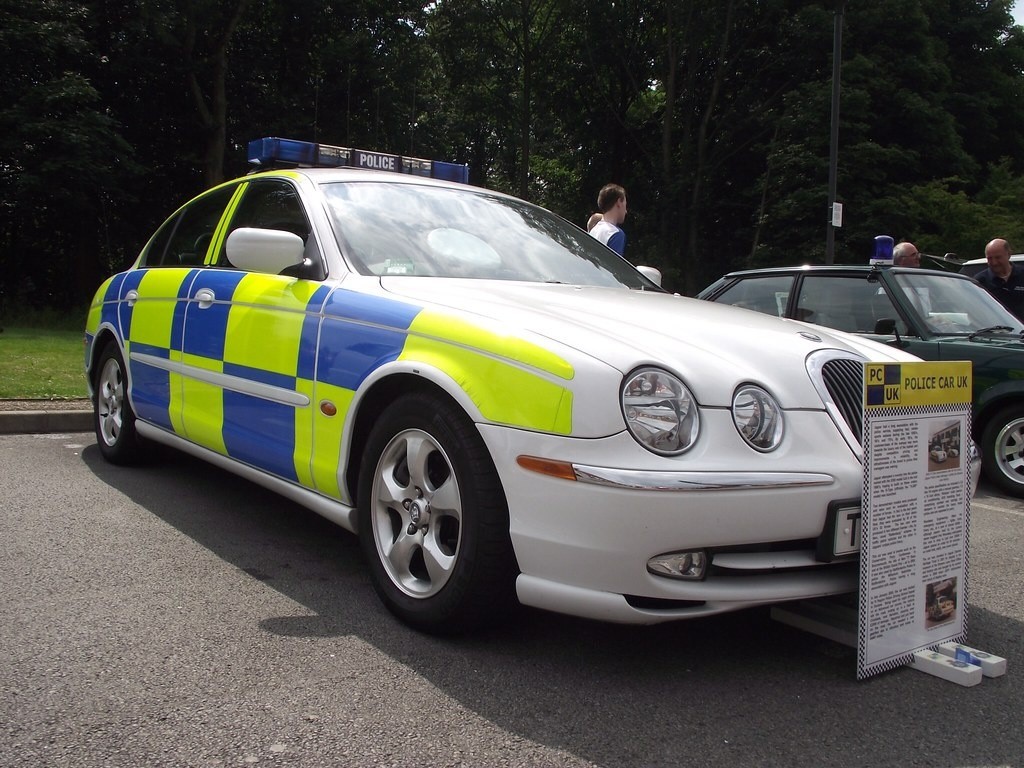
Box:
[973,239,1024,322]
[893,242,921,267]
[587,184,627,257]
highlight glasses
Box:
[900,252,922,259]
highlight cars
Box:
[687,235,1024,499]
[946,445,958,457]
[80,131,982,640]
[928,595,954,621]
[930,446,947,463]
